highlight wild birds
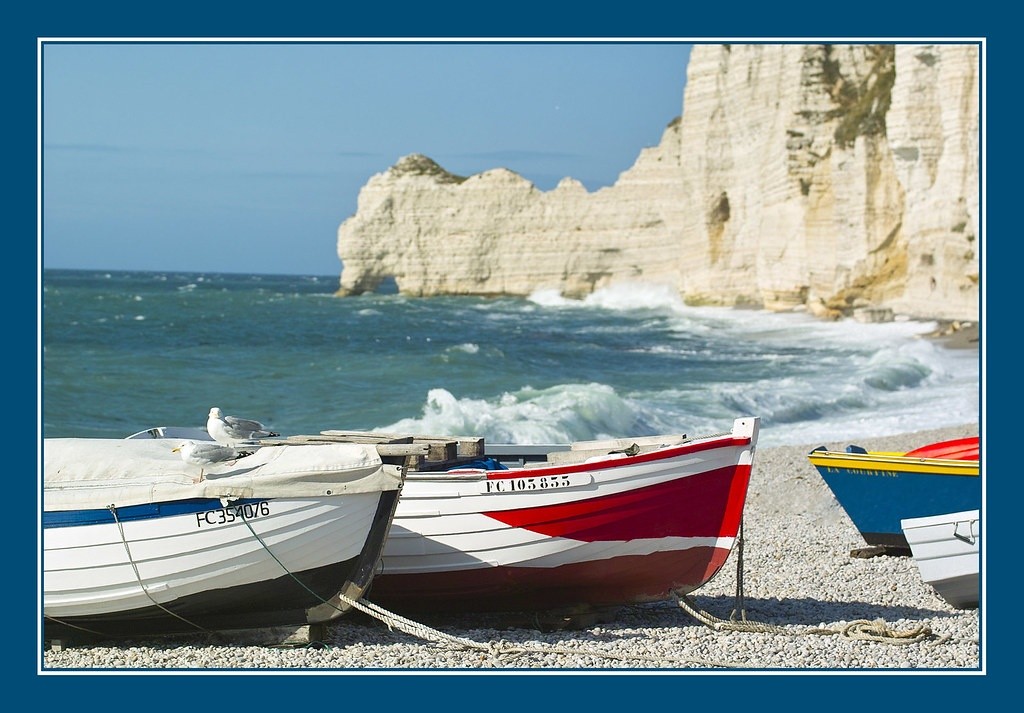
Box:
[207,407,280,442]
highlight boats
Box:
[41,436,413,627]
[343,416,759,613]
[809,437,981,549]
